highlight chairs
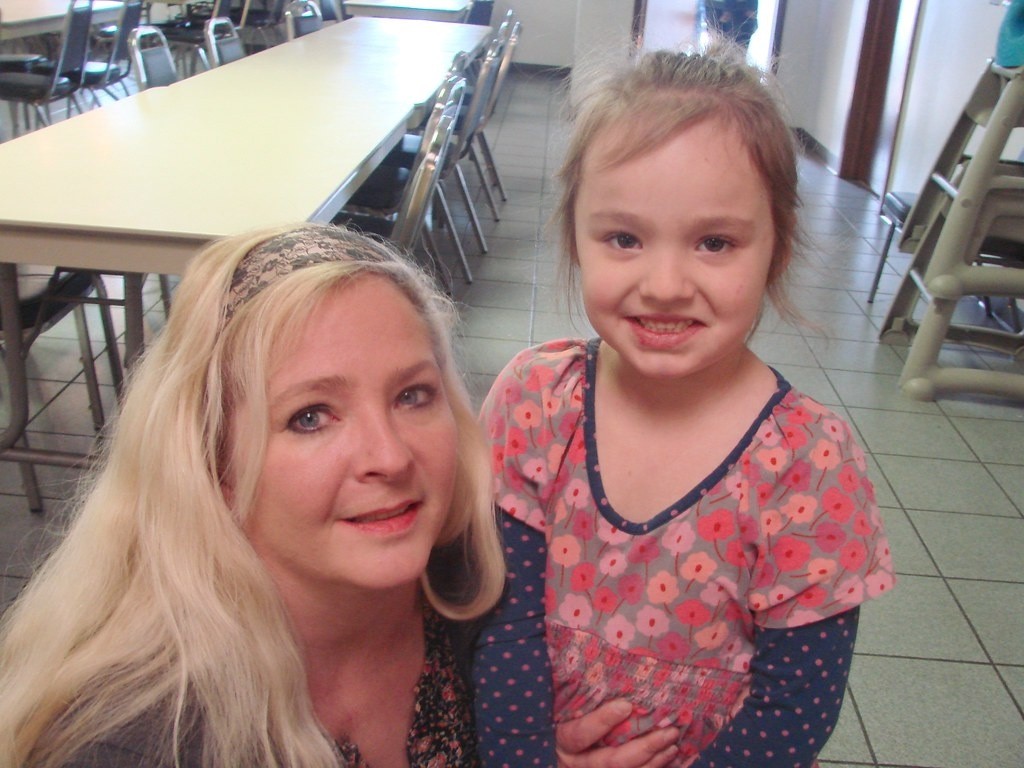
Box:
[873,0,1024,408]
[0,0,510,508]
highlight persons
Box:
[0,224,684,768]
[704,0,757,67]
[460,52,898,768]
